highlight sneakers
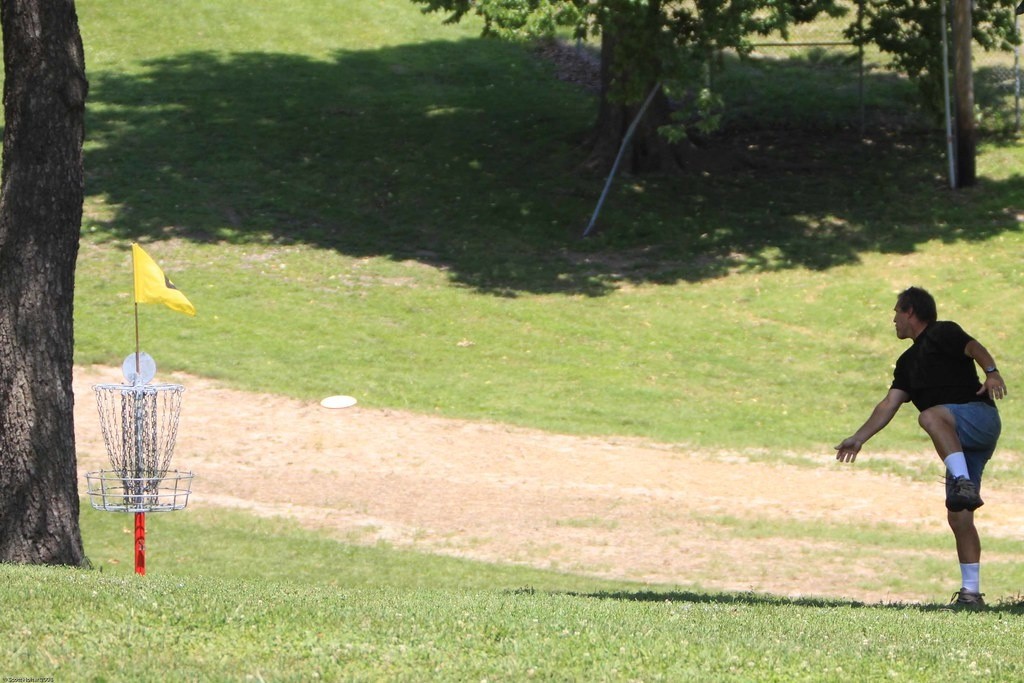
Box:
[938,475,984,512]
[940,587,985,613]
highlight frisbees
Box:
[321,395,358,409]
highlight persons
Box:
[835,287,1007,611]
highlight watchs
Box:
[984,365,999,374]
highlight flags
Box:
[132,242,196,317]
